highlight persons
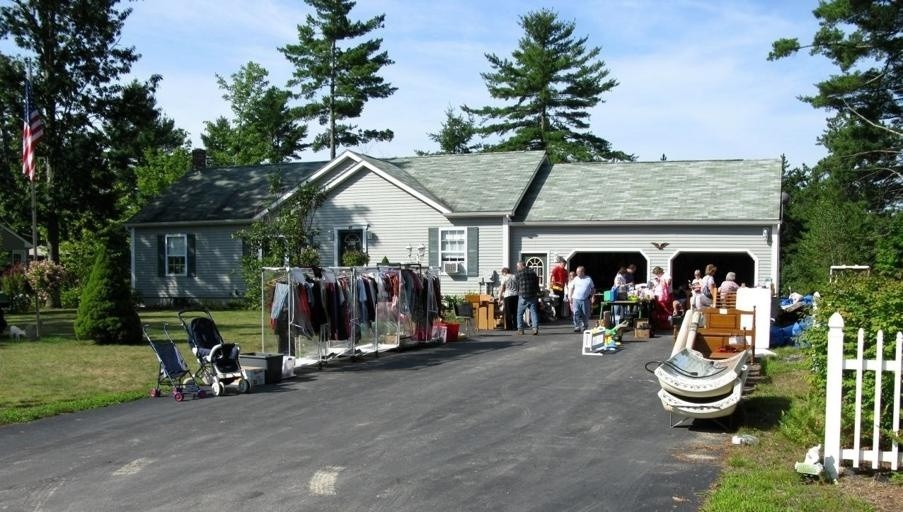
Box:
[568,270,575,325]
[513,261,539,336]
[498,267,519,331]
[567,265,596,333]
[550,260,569,320]
[610,264,718,344]
[717,271,740,308]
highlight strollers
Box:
[178,309,249,396]
[143,324,206,401]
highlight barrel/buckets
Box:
[437,322,460,342]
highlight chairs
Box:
[453,304,476,338]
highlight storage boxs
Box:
[432,322,459,343]
[238,351,296,389]
[635,318,651,338]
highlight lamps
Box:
[366,231,373,240]
[327,228,333,241]
[761,227,770,240]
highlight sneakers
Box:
[514,329,538,335]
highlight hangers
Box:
[267,268,438,287]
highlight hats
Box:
[705,264,716,275]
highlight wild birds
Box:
[651,243,669,250]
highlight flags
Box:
[21,58,44,182]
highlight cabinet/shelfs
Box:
[735,287,772,348]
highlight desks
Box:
[599,300,643,320]
